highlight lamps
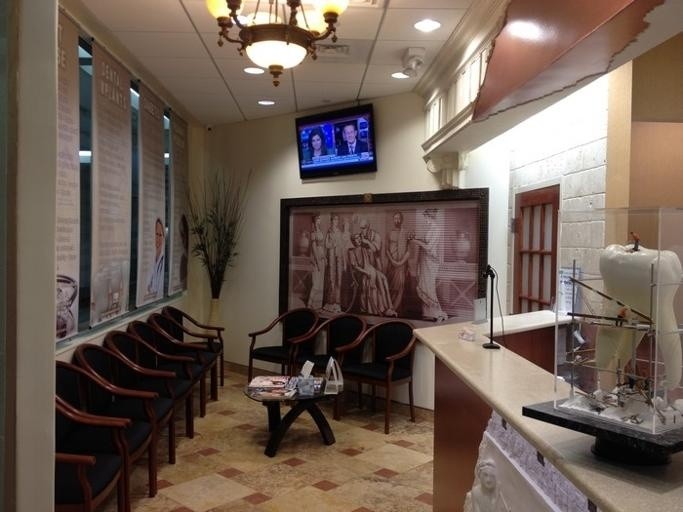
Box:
[206,0,351,78]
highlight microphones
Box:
[483,265,491,279]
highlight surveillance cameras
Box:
[206,123,213,133]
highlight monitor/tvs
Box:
[293,102,378,180]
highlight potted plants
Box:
[183,166,254,377]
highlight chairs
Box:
[56,305,224,512]
[248,308,416,434]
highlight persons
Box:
[463,458,505,512]
[307,214,327,313]
[348,219,399,318]
[337,123,368,156]
[308,126,328,160]
[382,210,411,315]
[147,219,165,296]
[411,207,448,322]
[325,213,347,313]
